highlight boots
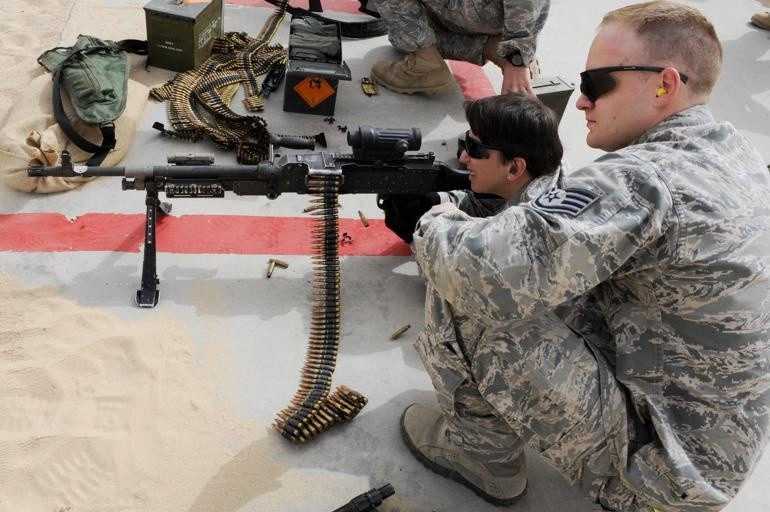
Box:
[370,43,456,96]
[483,34,540,80]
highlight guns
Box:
[27,126,505,308]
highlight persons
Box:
[356,0,551,101]
[401,1,770,512]
[750,10,770,31]
[383,92,580,288]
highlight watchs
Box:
[504,53,525,66]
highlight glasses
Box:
[580,66,689,102]
[464,129,502,160]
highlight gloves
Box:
[383,175,441,244]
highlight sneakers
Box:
[398,403,528,504]
